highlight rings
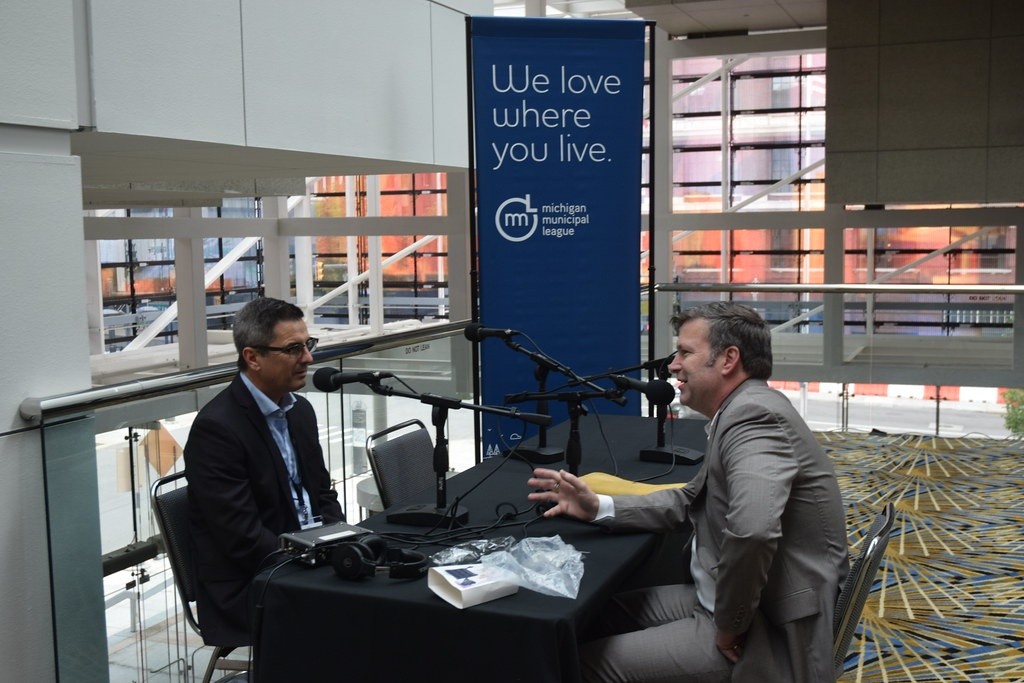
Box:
[554,483,560,490]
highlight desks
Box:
[254,416,712,683]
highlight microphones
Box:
[312,367,393,392]
[464,323,521,343]
[609,373,675,407]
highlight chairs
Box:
[366,418,437,510]
[148,469,256,683]
[828,502,894,681]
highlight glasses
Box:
[250,336,318,357]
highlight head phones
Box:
[332,534,430,583]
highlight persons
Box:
[182,297,346,648]
[527,301,852,683]
